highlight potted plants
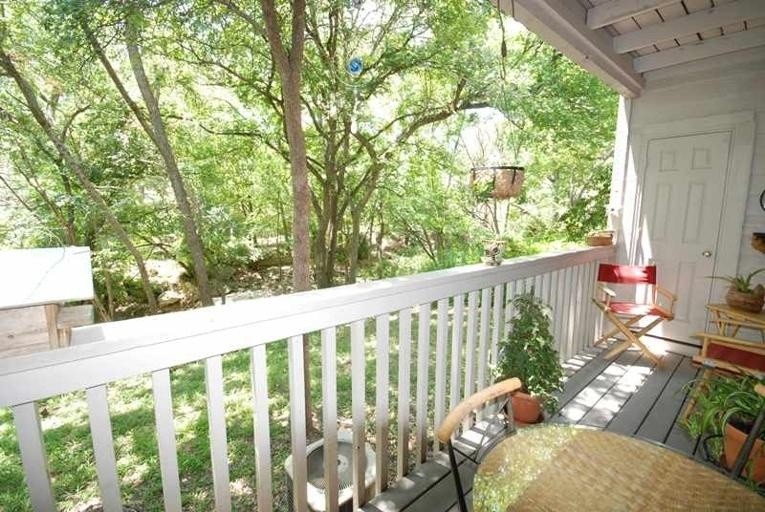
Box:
[469,166,528,204]
[679,361,765,494]
[492,286,565,423]
[702,266,765,312]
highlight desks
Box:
[471,419,765,512]
[707,302,764,347]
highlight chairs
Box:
[435,376,522,510]
[677,329,764,422]
[592,264,678,367]
